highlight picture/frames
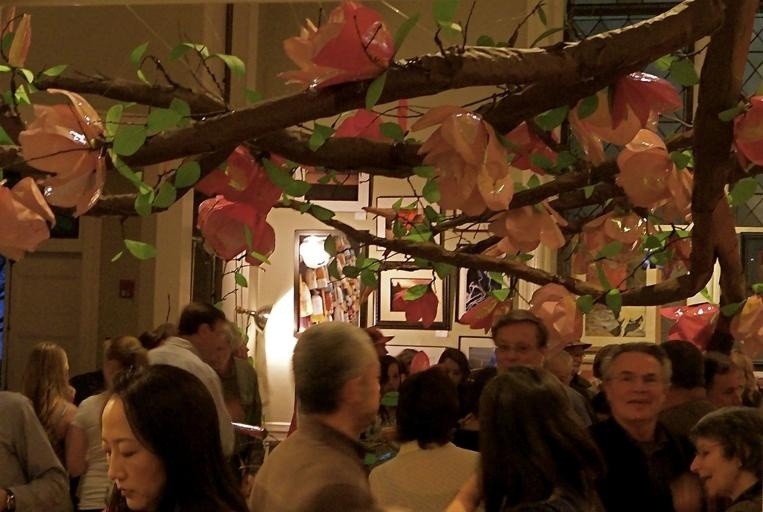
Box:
[580,268,663,346]
[294,166,518,339]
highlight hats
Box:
[564,334,592,351]
[367,326,395,345]
